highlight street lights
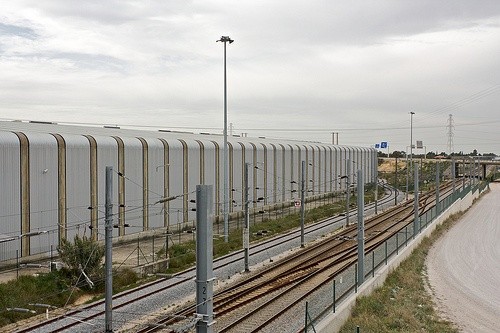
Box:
[216,35,235,242]
[409,111,415,169]
